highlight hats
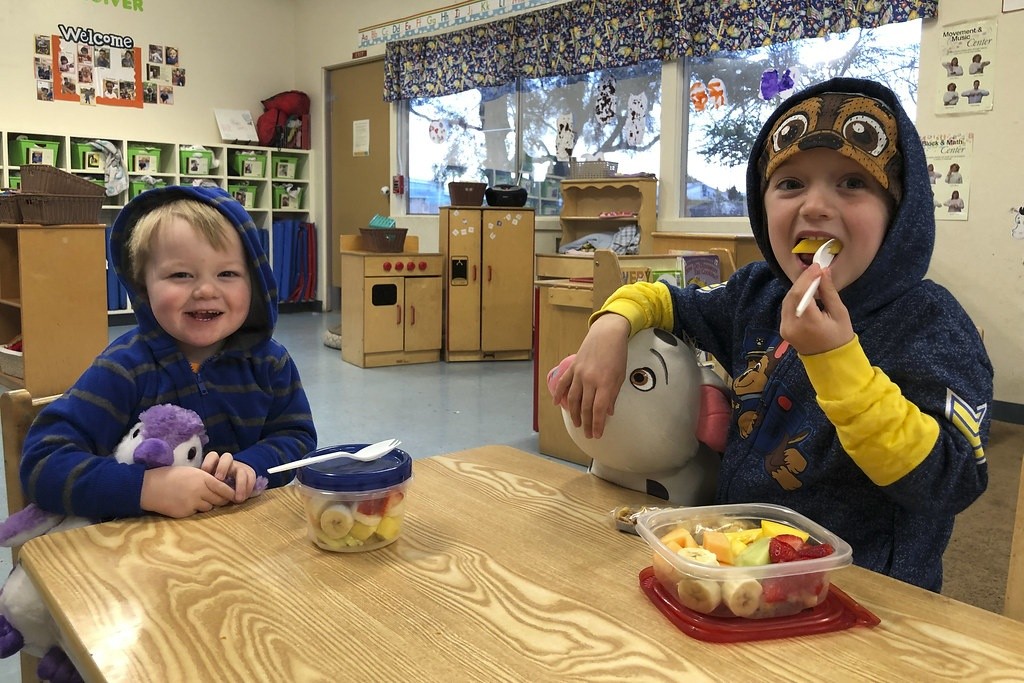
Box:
[758,92,902,208]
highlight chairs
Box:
[0,390,65,683]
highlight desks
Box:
[17,443,1024,683]
[650,230,764,282]
[536,252,594,279]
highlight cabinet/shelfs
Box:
[339,235,445,369]
[560,176,658,255]
[439,206,537,363]
[536,248,736,466]
[0,223,108,398]
[0,130,318,314]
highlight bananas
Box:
[674,547,763,617]
[303,495,404,538]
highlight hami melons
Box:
[658,528,734,580]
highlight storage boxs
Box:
[128,147,162,172]
[228,154,267,176]
[272,187,304,209]
[228,185,258,208]
[272,157,299,179]
[179,150,212,175]
[88,180,113,205]
[634,503,881,645]
[296,443,417,553]
[9,176,21,190]
[181,183,193,186]
[129,182,167,200]
[71,143,102,169]
[8,140,60,167]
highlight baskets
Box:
[564,160,619,180]
[449,182,487,207]
[359,214,408,252]
[16,165,107,223]
[0,194,23,224]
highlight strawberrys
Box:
[759,533,833,602]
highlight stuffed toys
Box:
[545,327,732,508]
[0,405,267,682]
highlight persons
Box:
[927,163,965,213]
[942,54,989,106]
[553,79,994,593]
[35,33,186,104]
[18,186,317,519]
